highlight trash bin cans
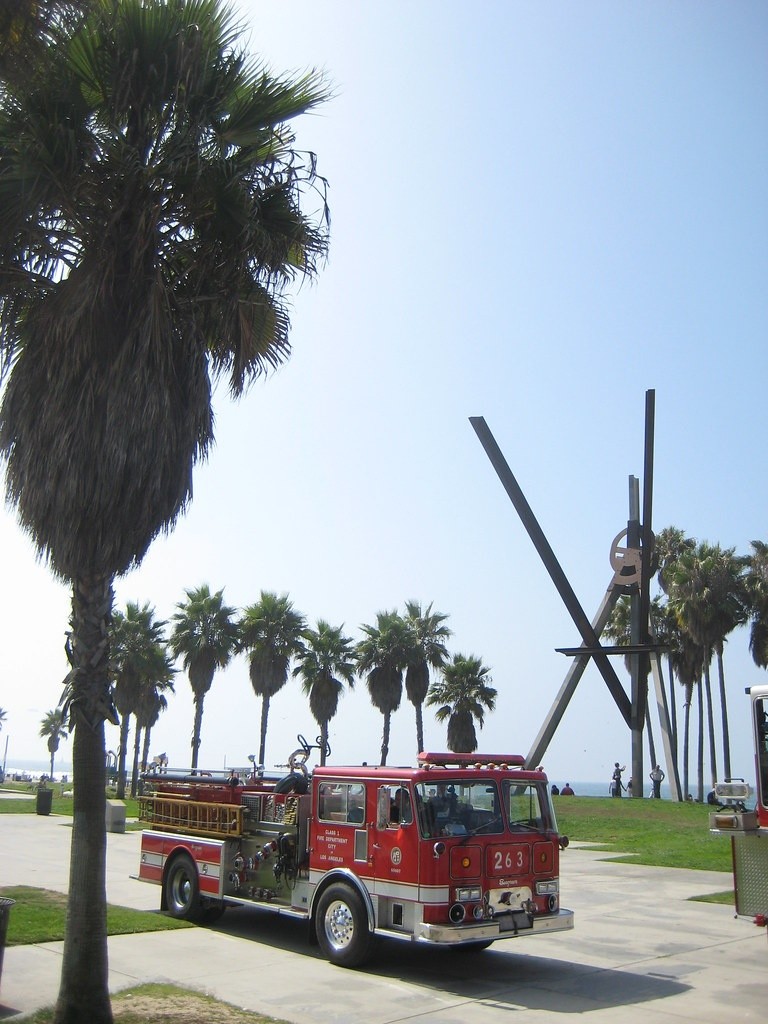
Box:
[36,787,54,816]
[0,896,16,986]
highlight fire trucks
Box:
[706,683,768,927]
[131,738,575,968]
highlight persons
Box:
[707,787,722,806]
[41,773,49,781]
[390,789,408,823]
[649,765,665,799]
[551,783,575,795]
[609,777,632,797]
